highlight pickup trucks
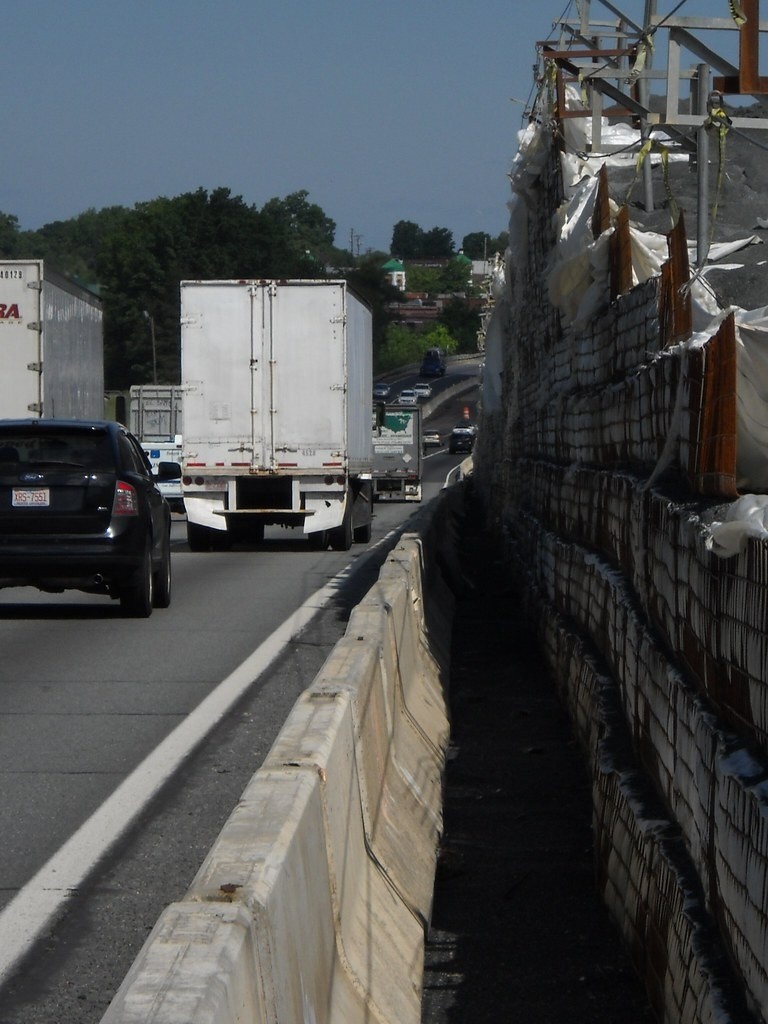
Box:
[136,435,187,515]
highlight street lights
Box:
[142,311,157,384]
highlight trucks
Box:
[179,280,386,551]
[372,404,428,503]
[0,258,105,421]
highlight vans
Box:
[424,348,446,364]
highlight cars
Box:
[449,424,479,455]
[412,383,433,400]
[1,419,183,617]
[373,383,391,399]
[420,360,445,379]
[396,390,418,404]
[422,429,442,448]
